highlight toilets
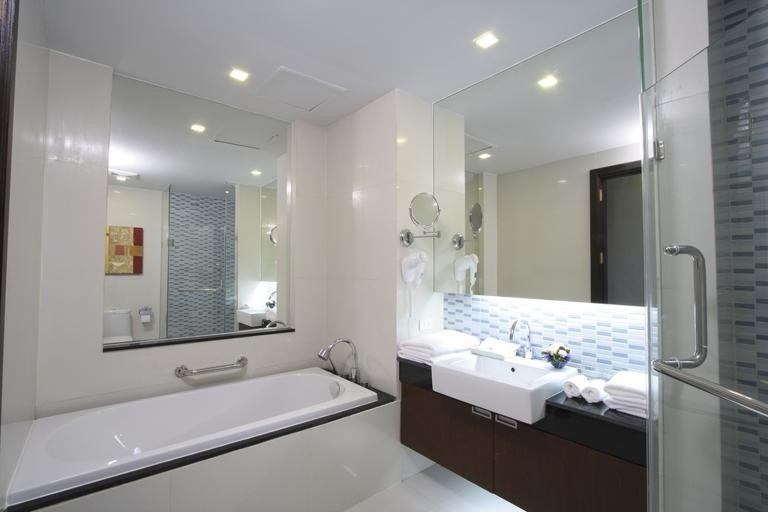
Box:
[103,309,134,344]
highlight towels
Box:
[562,371,659,421]
[398,329,520,366]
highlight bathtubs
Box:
[2,365,378,508]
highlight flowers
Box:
[541,343,571,368]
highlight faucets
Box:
[319,336,359,378]
[266,320,286,328]
[510,317,534,360]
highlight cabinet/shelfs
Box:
[400,382,647,512]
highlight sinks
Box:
[430,349,579,427]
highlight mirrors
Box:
[103,73,295,352]
[432,1,658,308]
[452,202,483,249]
[399,192,441,247]
[259,177,278,282]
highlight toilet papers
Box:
[139,307,151,324]
